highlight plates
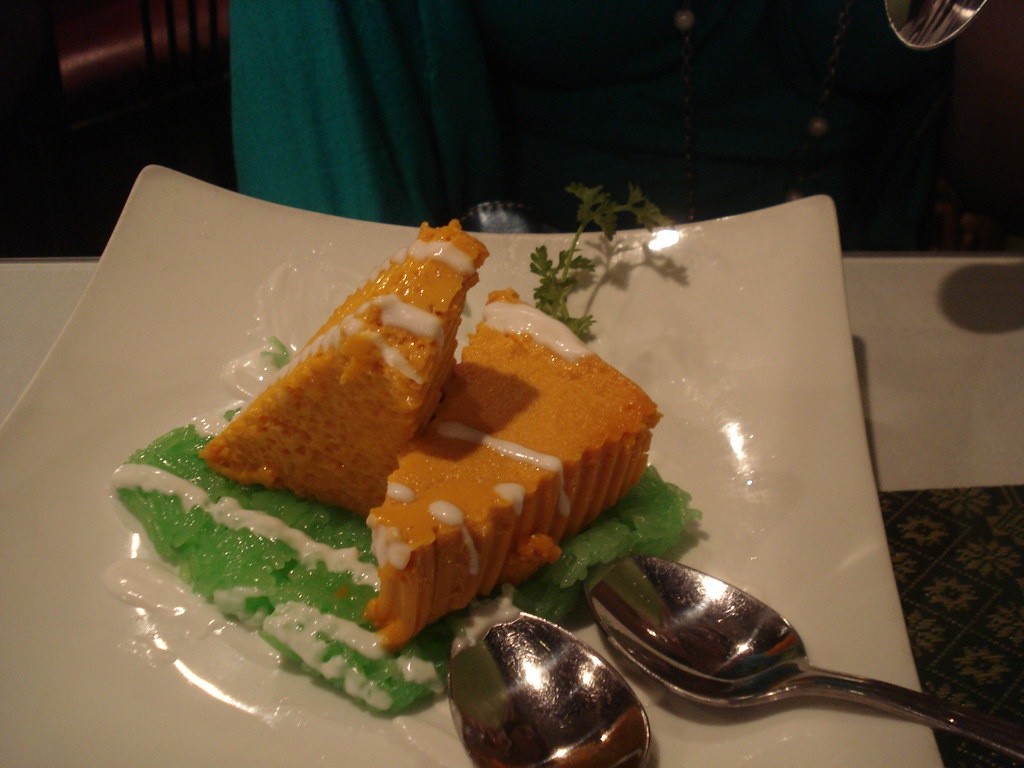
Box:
[1,163,942,767]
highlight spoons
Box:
[885,1,985,48]
[445,607,652,768]
[586,555,1023,763]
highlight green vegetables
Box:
[530,180,674,342]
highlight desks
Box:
[0,255,1024,768]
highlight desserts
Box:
[110,219,705,718]
